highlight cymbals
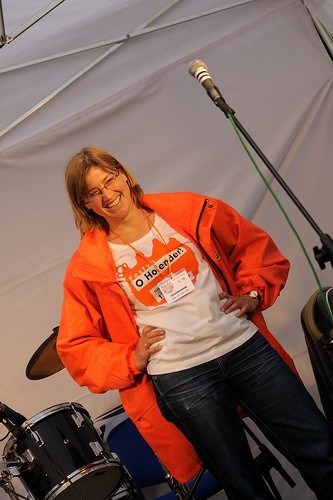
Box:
[25,326,66,380]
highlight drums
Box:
[1,400,128,500]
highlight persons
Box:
[58,147,333,500]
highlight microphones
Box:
[188,59,231,118]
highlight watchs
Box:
[247,290,262,303]
